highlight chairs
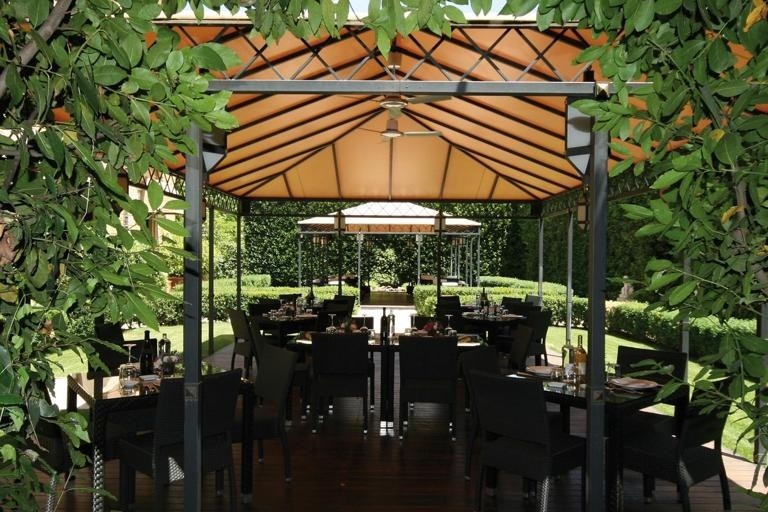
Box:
[215,343,294,487]
[457,346,504,495]
[467,364,589,510]
[115,368,242,512]
[226,295,554,445]
[88,338,157,372]
[614,346,689,382]
[22,380,94,512]
[605,375,735,510]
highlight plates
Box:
[610,376,657,390]
[526,365,552,378]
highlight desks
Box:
[460,311,528,339]
[285,330,484,417]
[506,366,668,500]
[67,362,255,512]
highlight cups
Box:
[475,294,511,319]
[263,297,323,320]
[564,369,576,383]
[551,367,563,380]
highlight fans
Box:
[341,53,452,109]
[360,109,441,136]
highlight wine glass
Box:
[360,313,369,332]
[326,313,337,333]
[410,313,418,334]
[122,344,137,383]
[443,314,454,335]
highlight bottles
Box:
[158,333,172,356]
[380,306,395,338]
[307,285,316,306]
[481,287,488,311]
[139,329,154,374]
[561,339,574,368]
[574,336,586,375]
[380,338,395,346]
